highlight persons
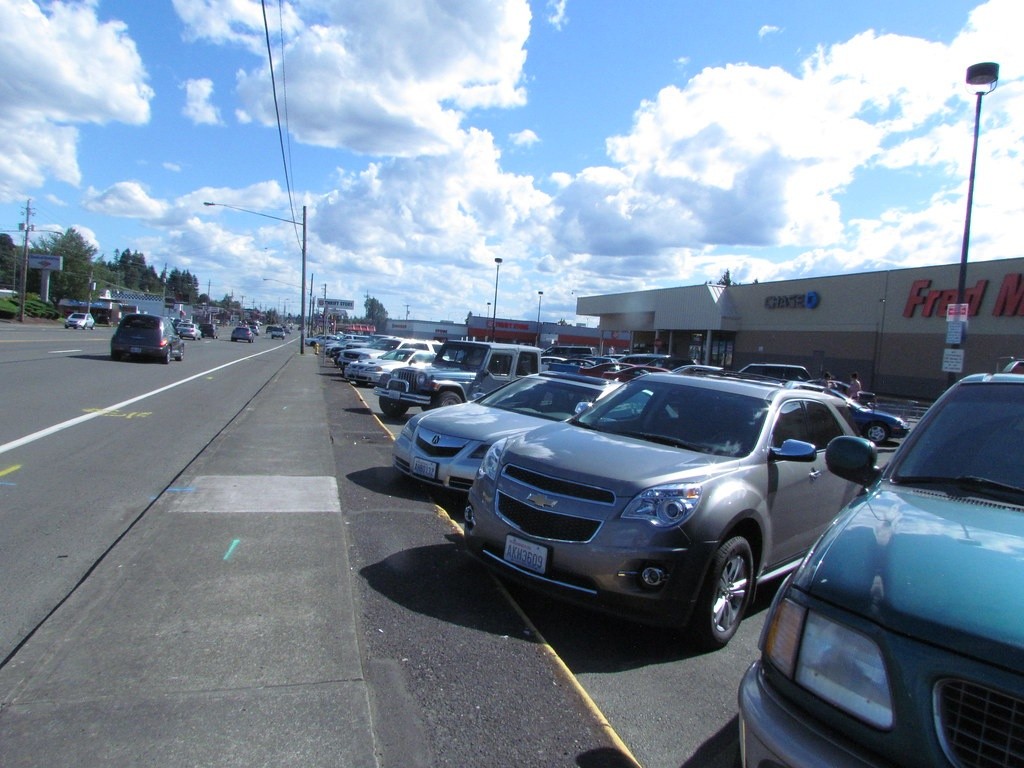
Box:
[609,345,615,353]
[847,373,861,399]
[824,372,837,389]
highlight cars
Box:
[266,323,301,340]
[169,317,192,329]
[541,347,911,445]
[463,372,866,652]
[392,371,679,501]
[65,313,95,330]
[737,373,1023,768]
[199,323,219,339]
[177,323,201,341]
[249,325,260,336]
[231,326,255,343]
[111,313,185,364]
[305,331,443,384]
[98,314,111,325]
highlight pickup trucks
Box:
[374,340,541,418]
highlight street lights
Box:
[491,258,502,343]
[487,302,491,342]
[535,291,543,347]
[204,202,307,355]
[943,63,999,390]
[264,273,313,338]
